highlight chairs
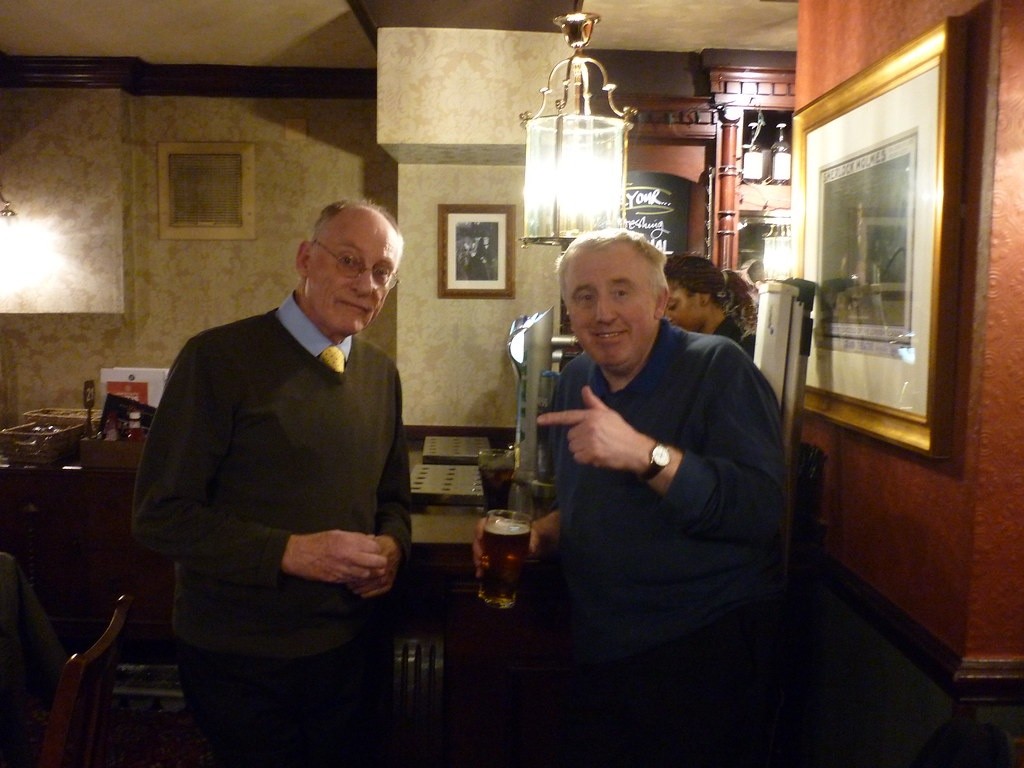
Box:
[35,594,132,768]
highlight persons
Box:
[470,228,799,768]
[132,196,411,768]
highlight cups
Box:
[475,509,533,609]
[477,448,515,517]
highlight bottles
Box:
[771,123,791,186]
[743,121,764,184]
[102,405,146,442]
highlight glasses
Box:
[313,238,401,290]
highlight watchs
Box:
[636,441,670,481]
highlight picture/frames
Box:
[437,202,518,302]
[790,17,977,461]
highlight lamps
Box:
[519,10,637,248]
[0,190,16,220]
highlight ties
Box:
[318,344,345,373]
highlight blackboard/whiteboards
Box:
[567,170,695,340]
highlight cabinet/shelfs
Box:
[0,468,176,642]
